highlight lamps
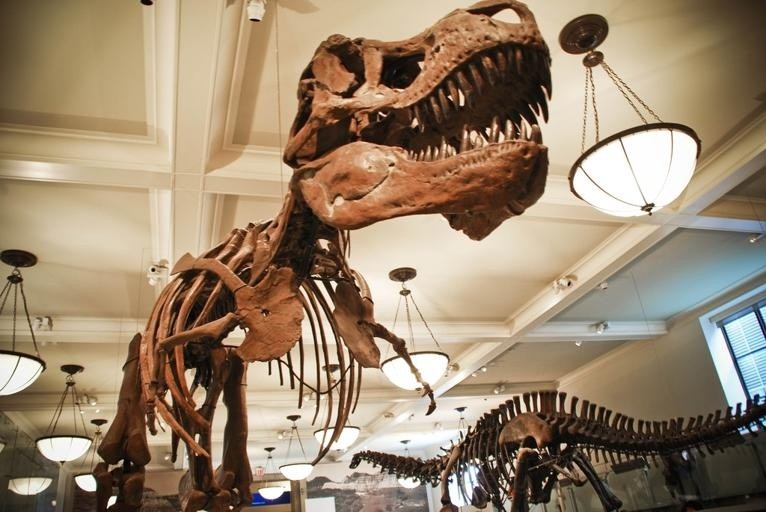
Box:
[0,249,109,498]
[556,13,704,221]
[249,364,365,505]
[380,266,451,395]
